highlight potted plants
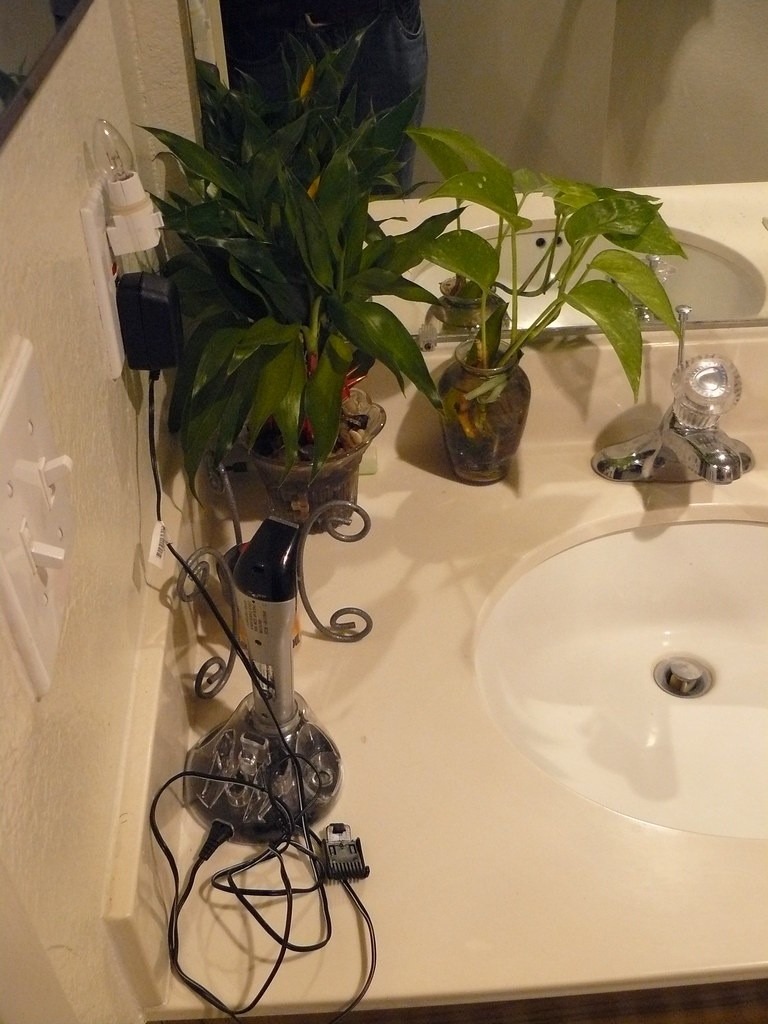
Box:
[404,126,662,337]
[403,116,692,483]
[114,14,432,539]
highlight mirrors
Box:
[180,0,768,353]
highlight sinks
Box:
[412,218,766,329]
[471,502,768,839]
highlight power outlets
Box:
[81,170,132,386]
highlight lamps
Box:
[91,116,165,262]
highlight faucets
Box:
[604,255,672,321]
[592,355,753,483]
